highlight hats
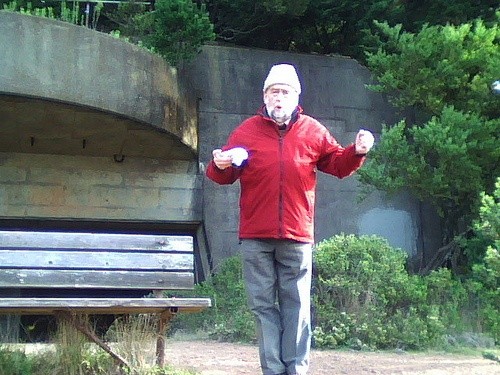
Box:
[262,64,302,95]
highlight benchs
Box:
[0,230,213,375]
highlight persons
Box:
[200,60,377,375]
